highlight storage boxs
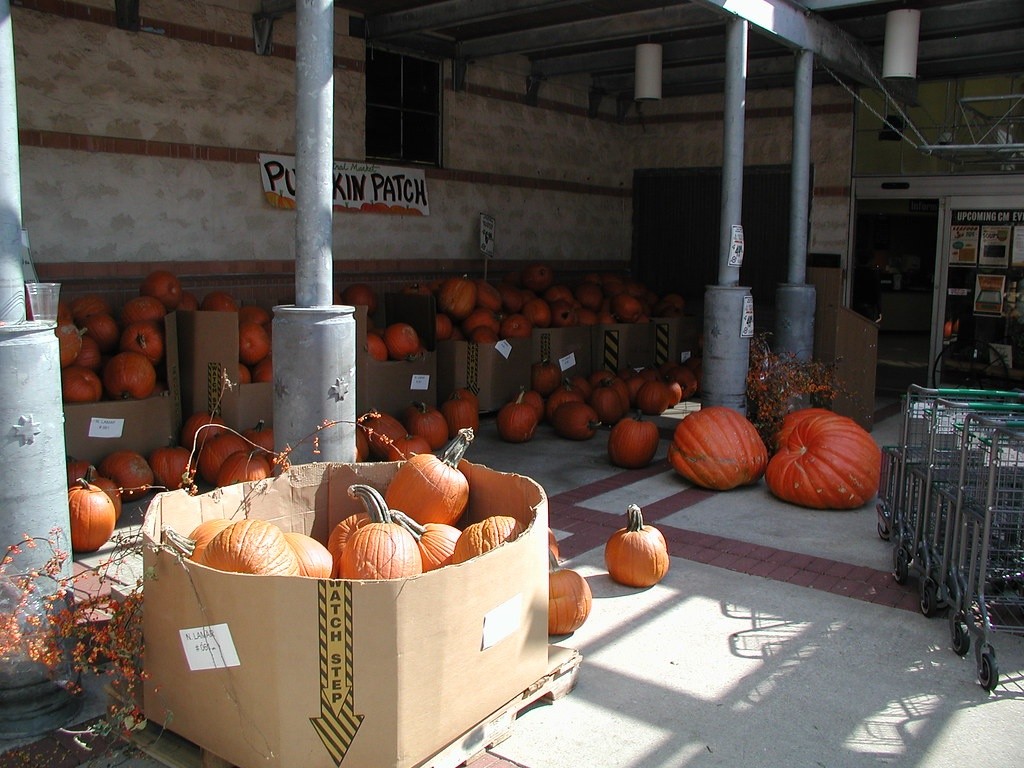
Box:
[63,308,703,463]
[143,458,549,767]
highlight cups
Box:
[25,282,60,323]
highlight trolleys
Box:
[876,382,1024,693]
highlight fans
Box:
[932,340,1009,402]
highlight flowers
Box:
[747,327,873,456]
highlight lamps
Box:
[879,94,909,141]
[634,37,663,102]
[882,9,921,80]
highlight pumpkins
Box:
[44,267,274,551]
[160,426,528,583]
[335,261,887,508]
[540,504,669,634]
